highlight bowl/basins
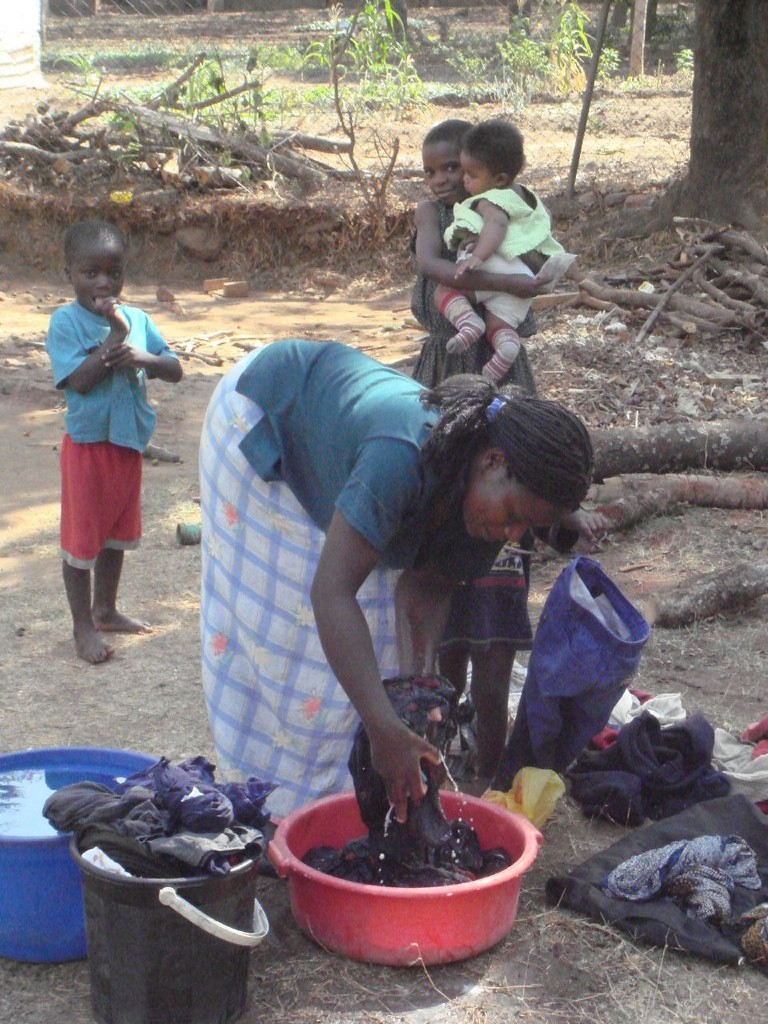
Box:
[265,789,544,965]
[0,749,179,964]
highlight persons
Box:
[433,120,565,384]
[411,121,555,404]
[47,220,182,664]
[423,373,607,796]
[200,337,608,875]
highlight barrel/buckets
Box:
[67,831,270,1024]
